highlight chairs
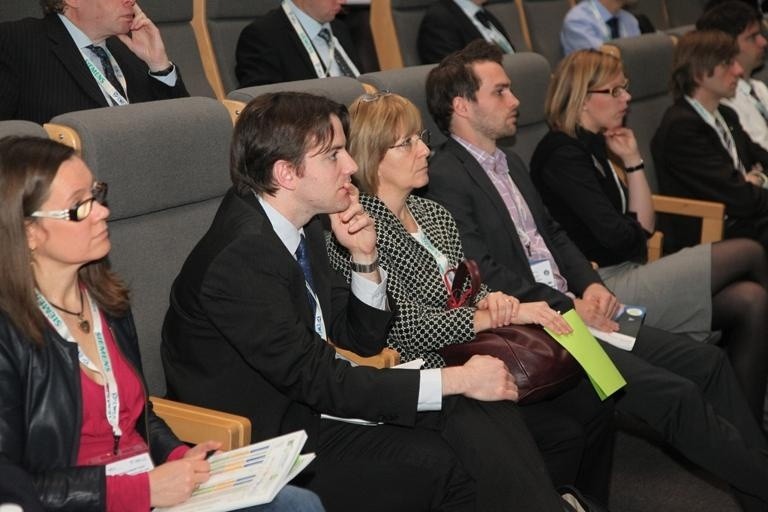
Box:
[1,1,768,453]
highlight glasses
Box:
[30,182,110,220]
[588,77,630,96]
[353,90,386,117]
[386,130,429,149]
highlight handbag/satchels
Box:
[441,258,583,404]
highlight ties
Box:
[475,10,489,27]
[606,16,620,40]
[319,29,356,79]
[87,43,127,105]
[296,237,314,293]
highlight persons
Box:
[327,87,616,511]
[234,0,365,87]
[162,89,576,511]
[418,1,768,512]
[2,1,192,126]
[0,135,325,512]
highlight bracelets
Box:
[150,65,173,75]
